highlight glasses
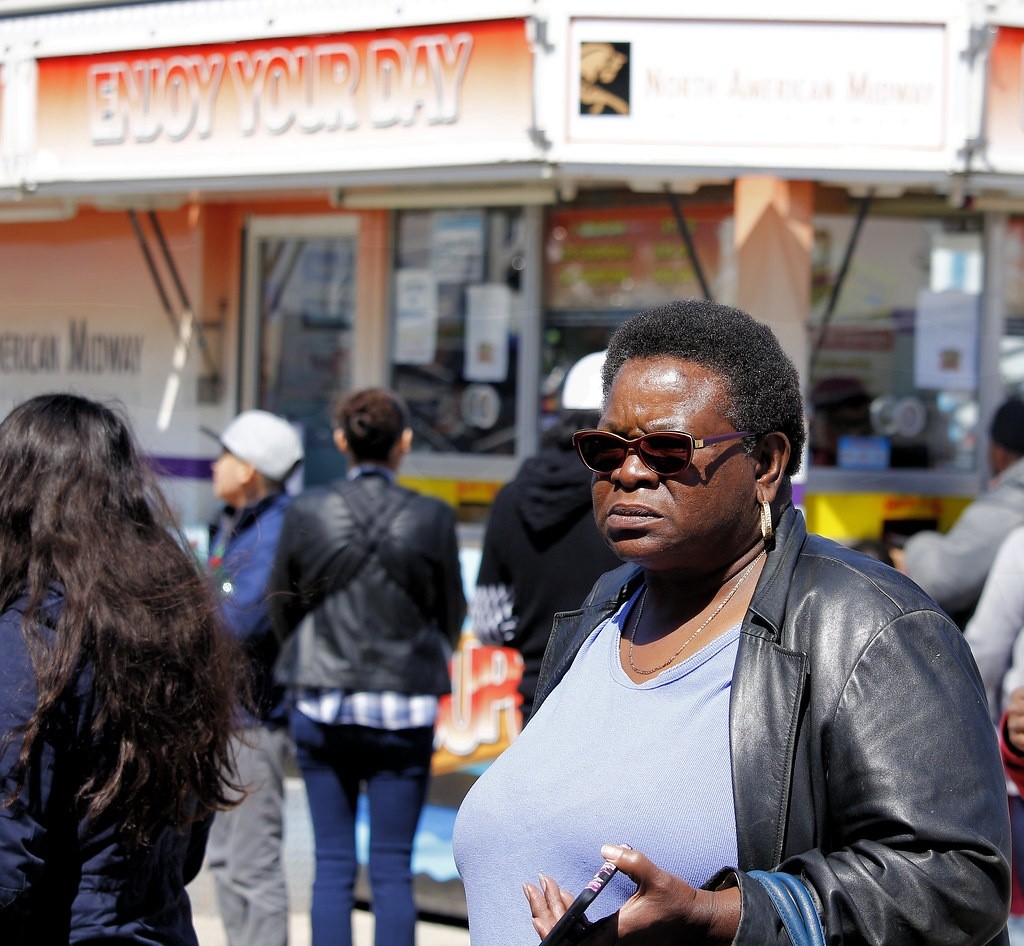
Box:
[571,428,752,472]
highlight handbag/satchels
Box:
[234,615,289,724]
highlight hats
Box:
[814,378,874,408]
[563,350,611,407]
[201,410,305,485]
[988,399,1024,455]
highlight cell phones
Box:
[538,843,632,946]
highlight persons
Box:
[199,409,306,946]
[272,384,469,946]
[890,392,1023,613]
[460,346,627,731]
[0,395,255,946]
[962,523,1023,946]
[451,298,1013,946]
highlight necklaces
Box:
[628,544,775,675]
